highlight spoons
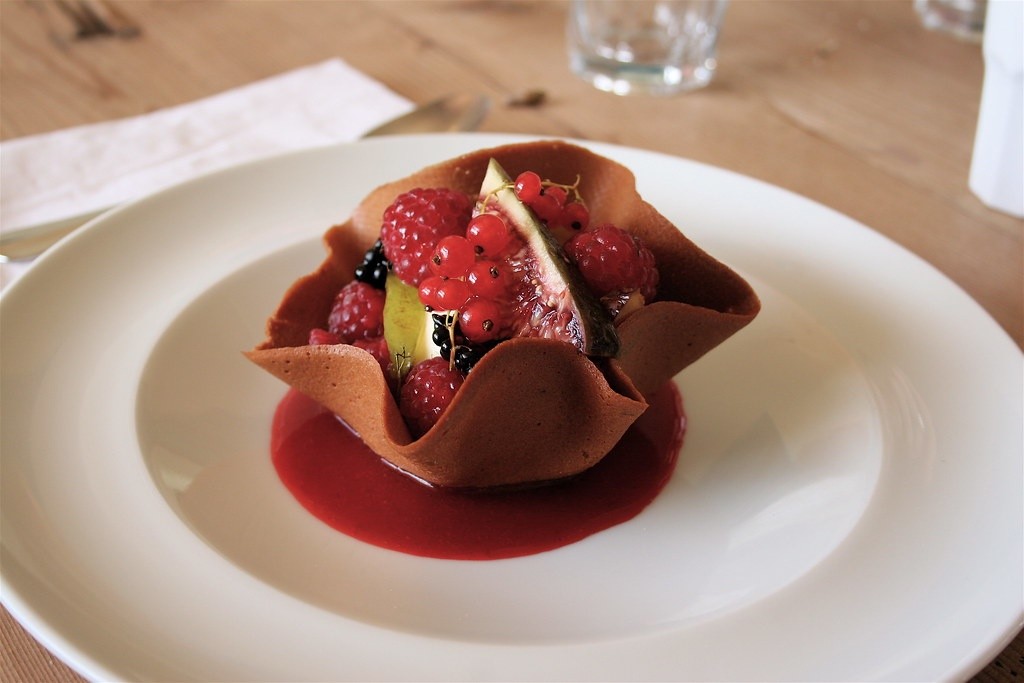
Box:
[0,94,497,265]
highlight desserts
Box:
[241,139,762,490]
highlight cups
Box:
[916,0,990,43]
[969,0,1024,221]
[567,0,729,97]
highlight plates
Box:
[0,132,1024,683]
